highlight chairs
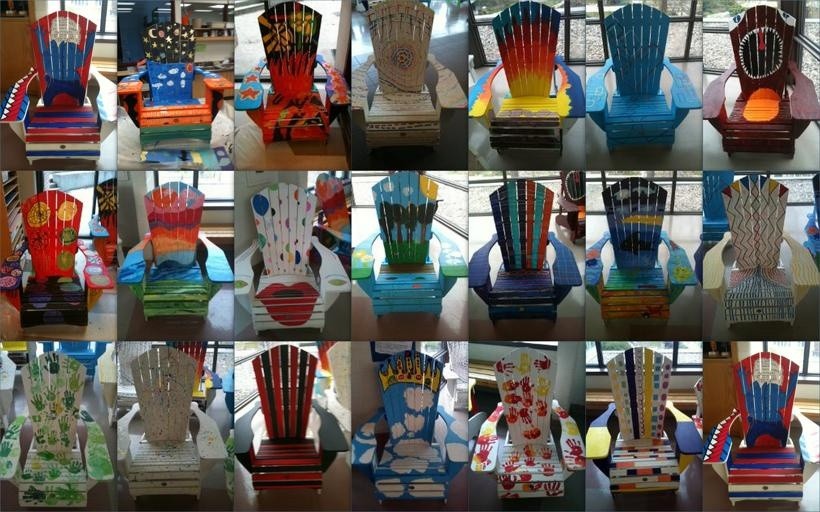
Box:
[0,2,820,511]
